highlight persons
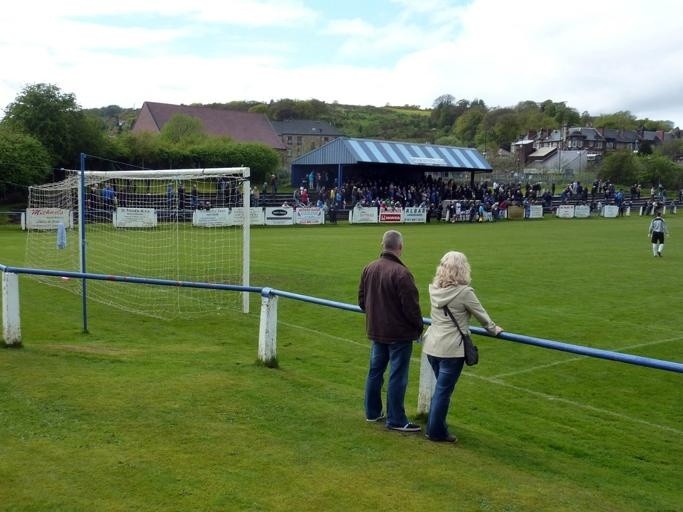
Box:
[561,178,676,217]
[294,171,554,219]
[356,230,423,433]
[422,249,505,444]
[88,174,277,210]
[646,211,669,258]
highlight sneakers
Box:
[425,433,458,443]
[366,412,385,422]
[653,251,664,257]
[386,421,421,432]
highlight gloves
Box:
[648,234,671,238]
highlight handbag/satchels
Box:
[463,337,481,367]
